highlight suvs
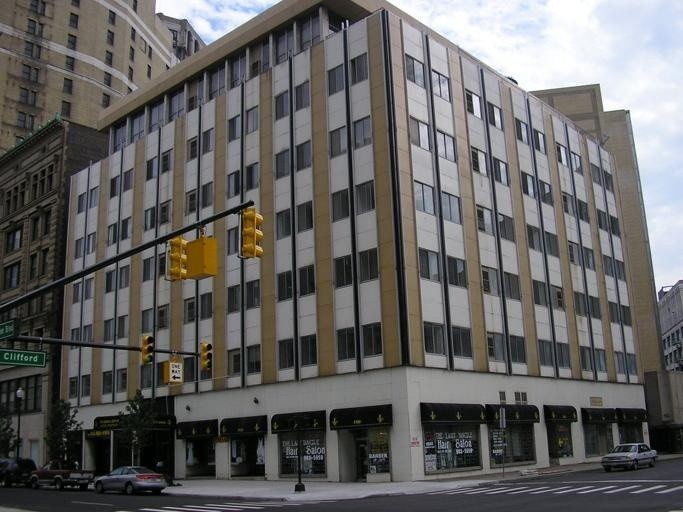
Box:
[0,456,38,487]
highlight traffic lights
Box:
[199,339,214,373]
[141,334,154,367]
[239,207,264,259]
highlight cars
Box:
[599,442,659,473]
[94,465,167,495]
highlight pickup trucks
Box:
[29,459,95,491]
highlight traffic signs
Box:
[0,348,47,368]
[169,362,184,383]
[167,235,188,282]
[0,318,19,342]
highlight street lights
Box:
[15,386,25,460]
